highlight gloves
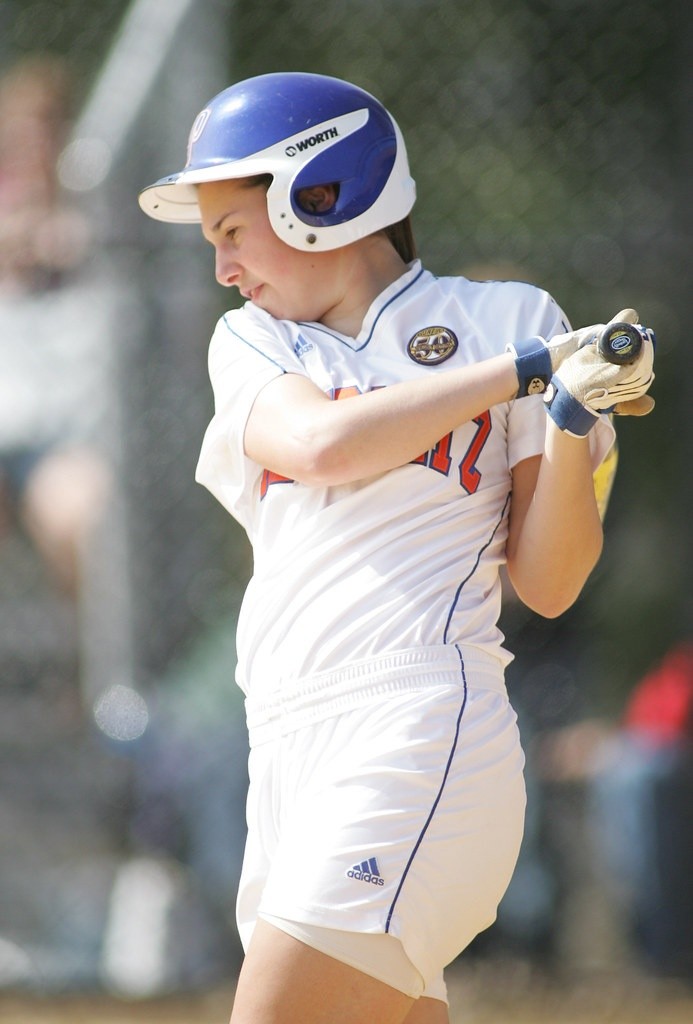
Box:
[541,308,656,439]
[505,324,607,399]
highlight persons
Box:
[138,72,655,1024]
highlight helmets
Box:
[135,71,418,253]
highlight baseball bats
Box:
[600,319,644,365]
